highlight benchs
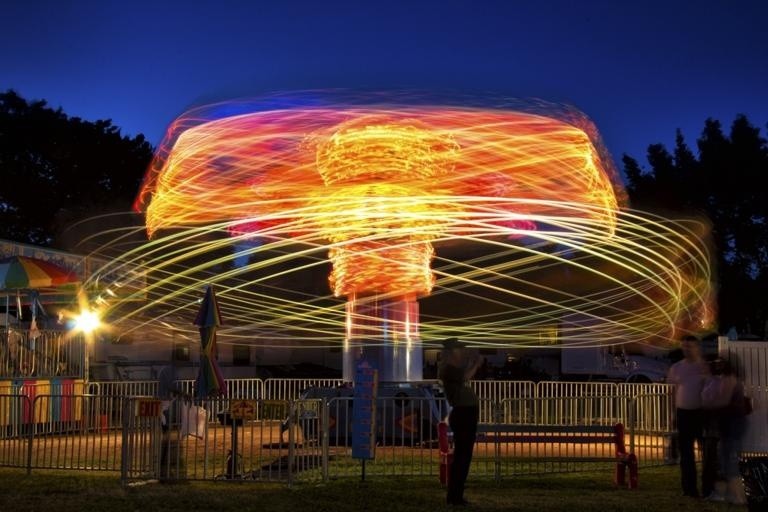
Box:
[436,421,639,488]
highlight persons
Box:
[156,345,193,486]
[704,359,755,508]
[436,336,487,504]
[700,351,744,409]
[664,332,716,499]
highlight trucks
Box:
[419,313,676,386]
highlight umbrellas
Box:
[191,283,228,480]
[0,255,80,327]
